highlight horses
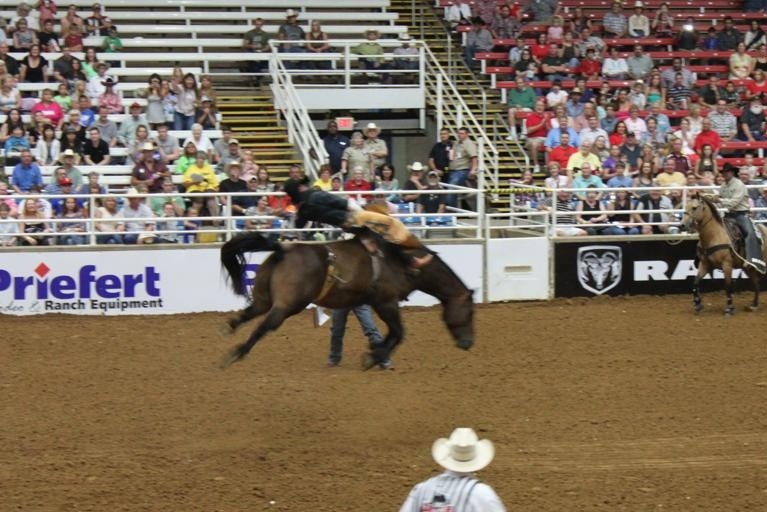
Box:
[680,190,767,317]
[216,230,475,370]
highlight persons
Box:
[436,1,767,235]
[239,8,420,91]
[705,160,757,273]
[1,1,298,247]
[325,198,396,371]
[288,122,478,239]
[283,172,436,269]
[394,423,512,512]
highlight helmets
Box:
[286,7,301,21]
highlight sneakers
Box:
[405,252,436,274]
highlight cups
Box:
[178,236,184,244]
[189,235,195,243]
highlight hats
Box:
[718,161,742,176]
[406,160,440,180]
[625,130,636,137]
[429,423,497,476]
[363,122,381,136]
[284,177,309,201]
[365,25,415,44]
[633,79,646,87]
[631,0,647,12]
[569,86,583,95]
[51,73,260,202]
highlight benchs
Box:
[0,2,419,233]
